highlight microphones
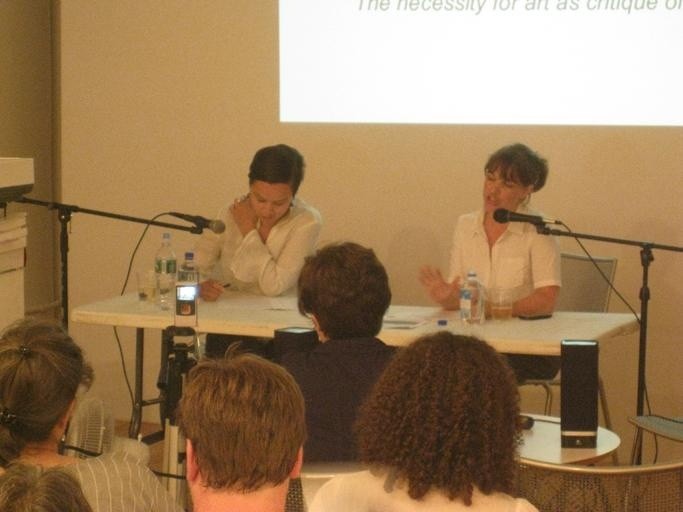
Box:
[493,209,560,229]
[173,212,226,235]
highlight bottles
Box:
[177,249,200,308]
[155,231,176,310]
[458,270,486,326]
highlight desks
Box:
[72,289,646,469]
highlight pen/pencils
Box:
[384,320,419,324]
[223,283,230,288]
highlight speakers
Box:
[558,339,599,448]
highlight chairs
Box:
[515,252,619,469]
[285,466,372,512]
[511,451,683,512]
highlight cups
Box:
[489,289,514,322]
[134,270,159,304]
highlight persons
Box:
[283,241,407,462]
[1,314,186,511]
[191,142,322,302]
[0,457,94,511]
[304,329,540,512]
[174,340,305,512]
[416,142,565,317]
